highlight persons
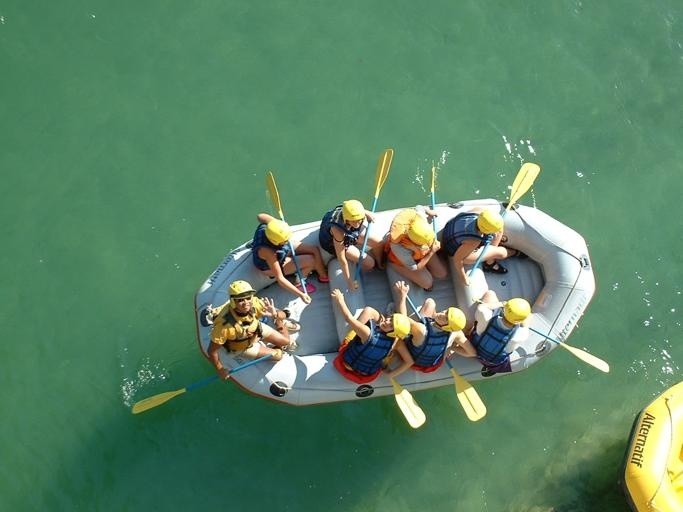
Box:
[470,290,533,370]
[332,289,416,385]
[250,211,330,302]
[394,281,477,373]
[208,282,302,382]
[380,206,446,291]
[447,205,530,288]
[318,198,385,292]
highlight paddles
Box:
[353,150,394,289]
[407,296,486,422]
[467,163,540,279]
[266,170,310,299]
[383,366,426,429]
[472,299,610,373]
[429,161,437,241]
[133,351,280,414]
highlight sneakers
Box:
[266,276,329,350]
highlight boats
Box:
[616,378,683,512]
[189,196,598,412]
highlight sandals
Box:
[483,261,508,273]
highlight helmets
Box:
[441,307,466,332]
[386,313,411,338]
[265,219,292,246]
[504,298,531,324]
[408,220,435,245]
[477,209,504,235]
[342,199,366,224]
[229,280,257,309]
